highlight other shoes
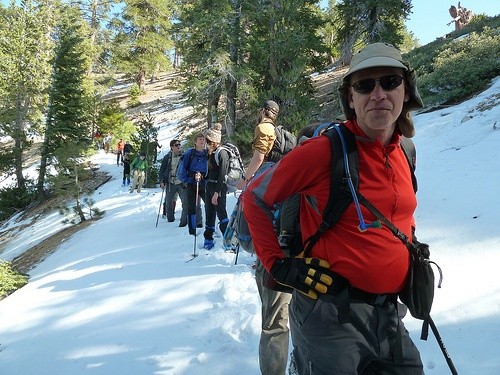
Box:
[203,239,214,250]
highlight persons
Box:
[153,139,162,164]
[159,100,328,374]
[241,42,442,375]
[95,129,152,194]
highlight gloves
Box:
[268,249,335,301]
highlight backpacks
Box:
[224,161,281,256]
[176,149,209,181]
[215,143,246,194]
[277,115,417,258]
[261,120,296,162]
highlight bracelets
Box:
[242,174,248,182]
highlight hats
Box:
[258,100,279,114]
[204,123,222,143]
[339,42,424,120]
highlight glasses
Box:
[350,75,406,94]
[174,144,181,147]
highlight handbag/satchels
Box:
[398,241,443,341]
